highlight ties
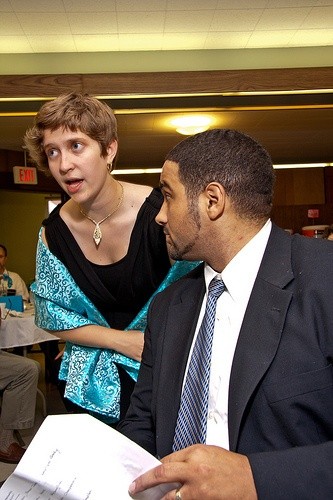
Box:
[172,279,226,453]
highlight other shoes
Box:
[0,442,26,464]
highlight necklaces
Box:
[78,180,124,247]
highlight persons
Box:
[120,129,333,500]
[0,245,66,384]
[25,91,203,432]
[0,350,42,464]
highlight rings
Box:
[176,488,181,500]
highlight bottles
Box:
[301,209,329,239]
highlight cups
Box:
[7,289,16,296]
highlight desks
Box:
[0,309,60,418]
